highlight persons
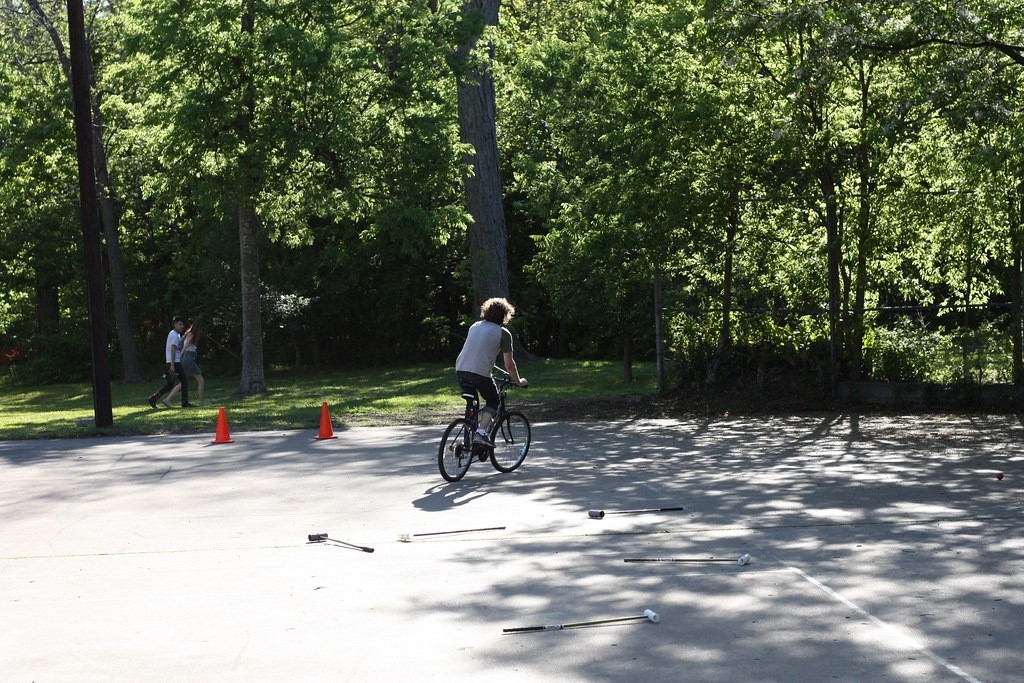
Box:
[162,319,210,409]
[455,298,528,448]
[148,316,194,408]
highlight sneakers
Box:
[472,431,497,448]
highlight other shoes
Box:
[199,402,213,408]
[163,399,172,408]
[181,401,194,407]
[147,394,157,409]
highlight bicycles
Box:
[437,364,532,482]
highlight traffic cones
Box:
[313,402,338,439]
[211,406,234,444]
